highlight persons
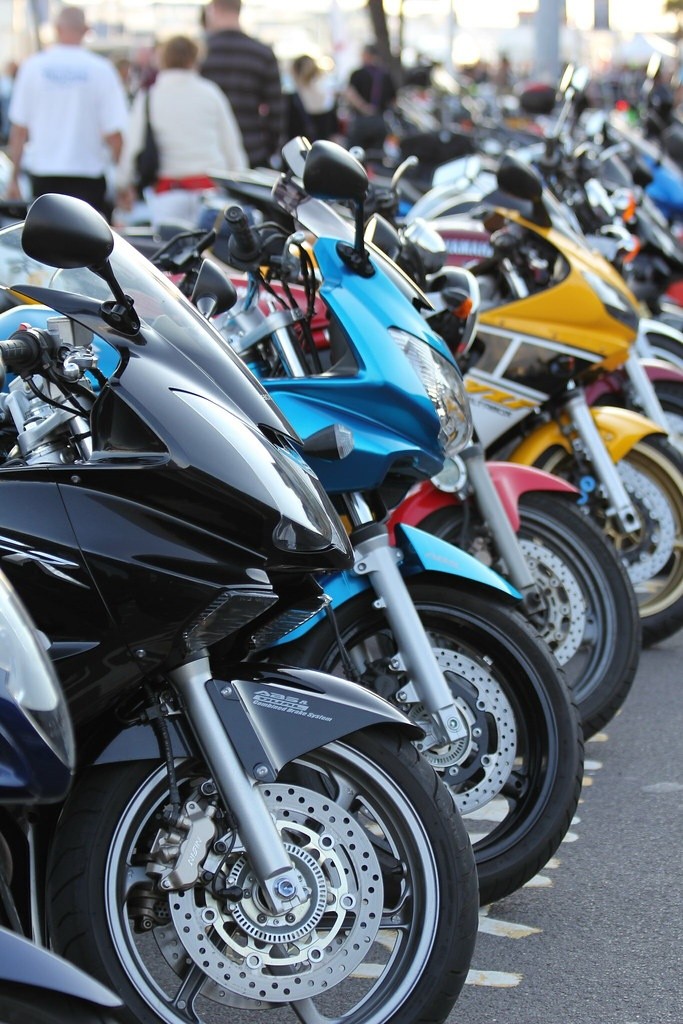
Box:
[344,45,397,167]
[283,55,342,147]
[196,0,285,169]
[117,36,250,227]
[4,8,133,226]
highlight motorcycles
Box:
[334,103,683,739]
[1,185,481,1024]
[1,577,131,1024]
[0,139,586,905]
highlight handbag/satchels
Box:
[135,86,160,199]
